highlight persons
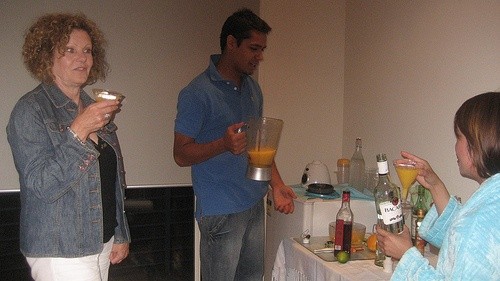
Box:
[374,91,500,281]
[172,8,299,281]
[5,11,133,281]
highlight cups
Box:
[92,87,122,119]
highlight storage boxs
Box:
[329,222,366,242]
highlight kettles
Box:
[301,159,330,189]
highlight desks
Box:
[272,236,437,281]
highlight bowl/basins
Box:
[329,221,366,251]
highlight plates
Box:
[309,184,334,194]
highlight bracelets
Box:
[66,125,87,146]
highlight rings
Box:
[105,114,110,120]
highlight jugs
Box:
[237,116,283,181]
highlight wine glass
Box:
[392,160,417,210]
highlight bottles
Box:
[375,236,393,274]
[414,209,425,256]
[337,158,350,183]
[349,138,365,193]
[373,153,405,235]
[334,191,353,256]
[410,185,428,255]
